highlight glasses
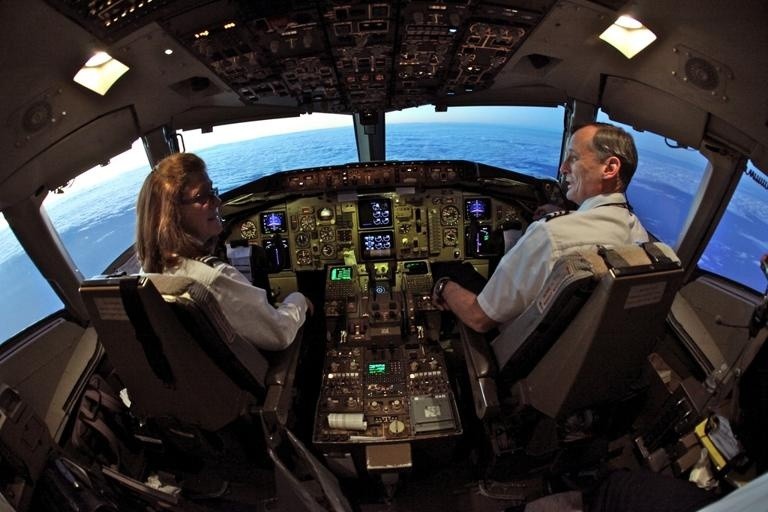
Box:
[180,188,217,204]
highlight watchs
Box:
[433,278,452,302]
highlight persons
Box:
[135,153,317,370]
[431,121,650,371]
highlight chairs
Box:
[78,270,311,497]
[454,240,685,488]
